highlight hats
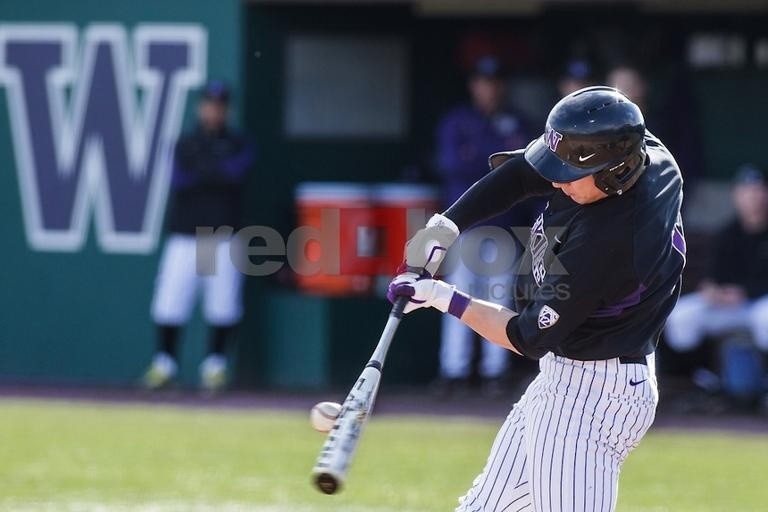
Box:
[562,61,589,81]
[198,80,232,103]
[725,163,764,187]
[463,56,507,82]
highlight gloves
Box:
[400,213,460,278]
[383,272,455,315]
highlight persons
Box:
[660,161,767,406]
[430,54,515,399]
[388,86,687,512]
[555,55,594,101]
[604,57,703,388]
[136,75,250,396]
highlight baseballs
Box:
[311,401,342,431]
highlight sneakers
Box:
[429,377,462,398]
[196,354,228,401]
[478,381,505,401]
[135,350,178,389]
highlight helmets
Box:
[522,84,649,195]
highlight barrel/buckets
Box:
[293,181,375,295]
[374,181,439,297]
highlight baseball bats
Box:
[310,294,409,496]
